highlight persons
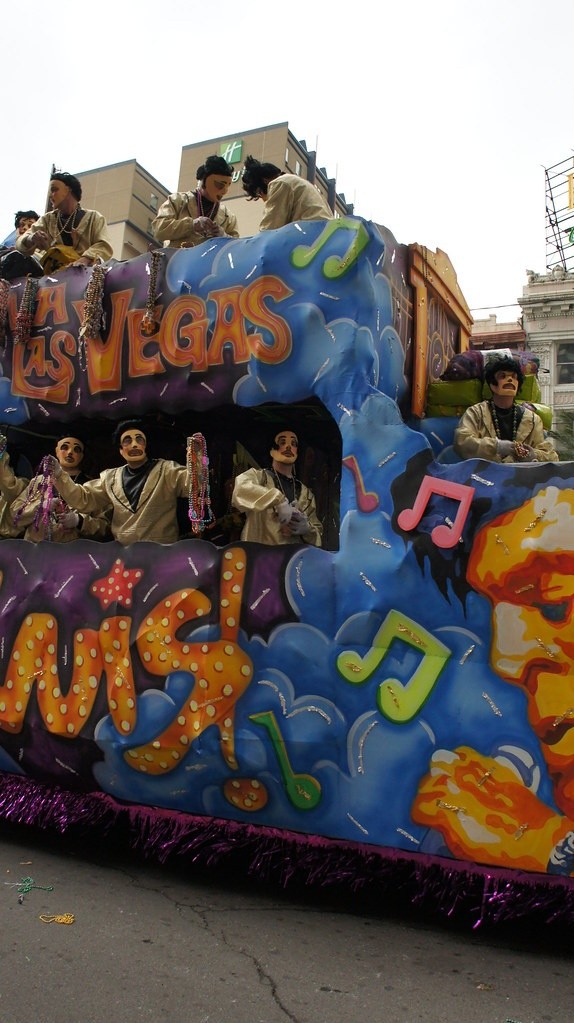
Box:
[455,360,561,462]
[240,158,335,234]
[0,210,48,276]
[42,419,218,547]
[10,432,106,544]
[150,155,239,246]
[14,171,110,268]
[0,433,32,540]
[231,427,325,550]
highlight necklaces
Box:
[225,453,248,523]
[0,434,8,459]
[13,454,72,542]
[185,433,217,534]
[139,249,166,336]
[78,263,106,375]
[0,279,10,345]
[487,396,518,449]
[509,437,532,461]
[52,205,79,238]
[54,468,84,506]
[193,189,217,237]
[271,465,297,503]
[14,278,37,346]
